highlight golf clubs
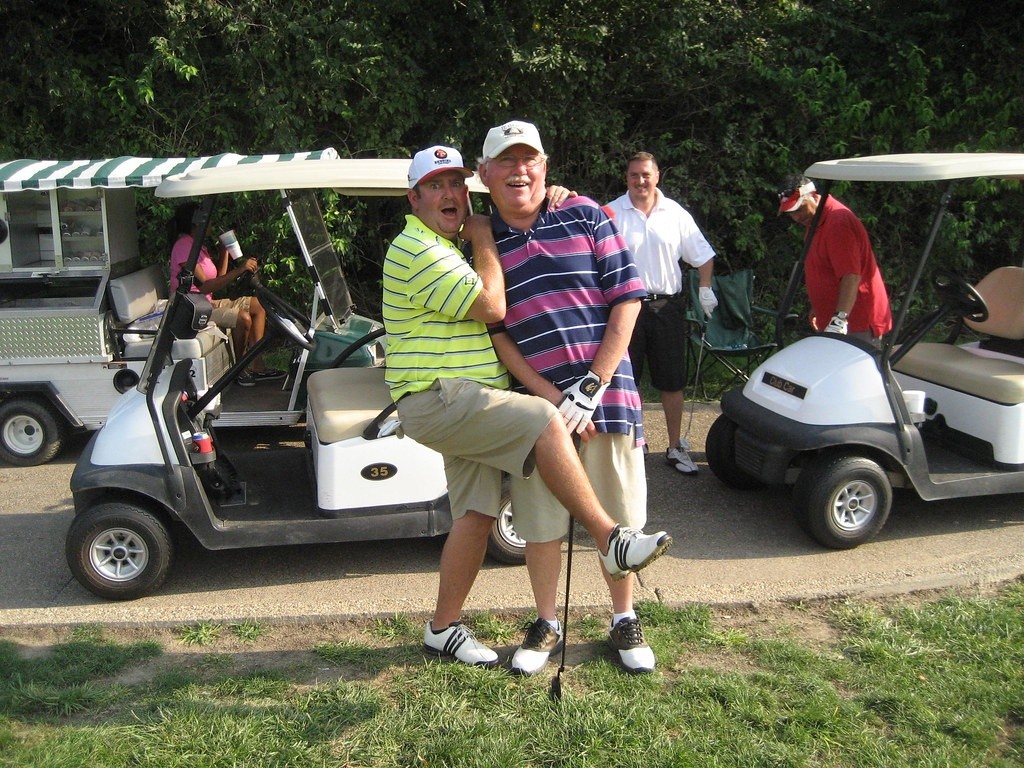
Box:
[680,313,710,452]
[550,430,584,699]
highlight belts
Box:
[398,391,412,400]
[643,291,680,301]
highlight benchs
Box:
[109,262,234,358]
[887,264,1023,403]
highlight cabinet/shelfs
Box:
[55,186,106,267]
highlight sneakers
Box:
[667,447,698,474]
[642,442,649,459]
[254,368,287,380]
[606,616,655,673]
[512,618,563,677]
[235,369,256,386]
[424,617,499,667]
[597,523,673,581]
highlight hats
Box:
[408,145,474,190]
[776,182,817,218]
[482,120,544,161]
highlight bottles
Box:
[723,344,748,350]
[193,437,212,455]
[61,219,73,230]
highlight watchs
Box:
[833,310,849,320]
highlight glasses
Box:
[487,154,548,168]
[778,185,804,200]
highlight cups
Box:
[197,390,216,409]
[903,390,926,415]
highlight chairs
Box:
[685,265,799,401]
[307,326,399,443]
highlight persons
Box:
[167,201,287,386]
[382,144,674,670]
[460,119,656,675]
[774,174,893,355]
[600,151,719,476]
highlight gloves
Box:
[823,316,848,335]
[556,370,610,436]
[699,286,718,319]
